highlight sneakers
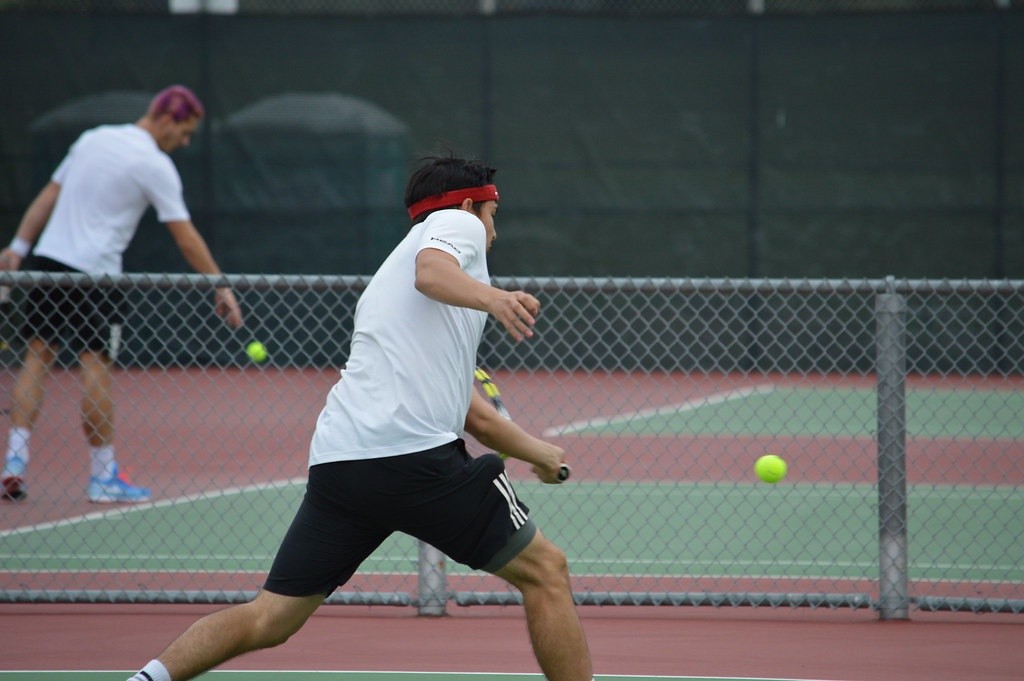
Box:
[0,453,29,497]
[87,465,152,503]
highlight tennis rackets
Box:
[0,255,10,351]
[464,364,571,481]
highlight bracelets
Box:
[9,239,31,258]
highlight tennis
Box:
[246,341,267,362]
[755,454,787,483]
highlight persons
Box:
[0,84,245,505]
[127,139,595,681]
